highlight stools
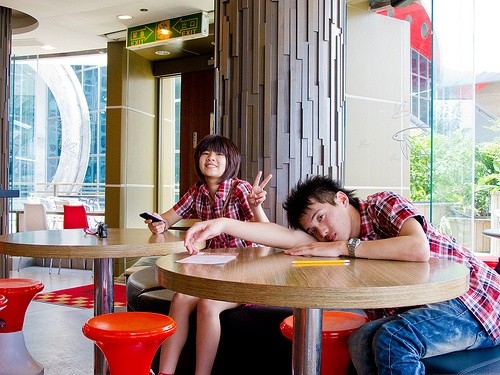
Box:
[279,312,369,375]
[81,312,177,375]
[0,278,45,375]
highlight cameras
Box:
[98,222,107,238]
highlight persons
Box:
[145,135,272,375]
[184,177,499,374]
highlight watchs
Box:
[347,237,361,258]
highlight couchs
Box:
[373,261,500,375]
[129,260,291,375]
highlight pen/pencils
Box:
[291,259,351,265]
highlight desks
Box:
[0,228,208,375]
[155,247,471,375]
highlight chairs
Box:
[17,203,62,275]
[58,204,96,278]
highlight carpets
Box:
[32,281,127,310]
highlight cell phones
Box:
[139,212,165,223]
